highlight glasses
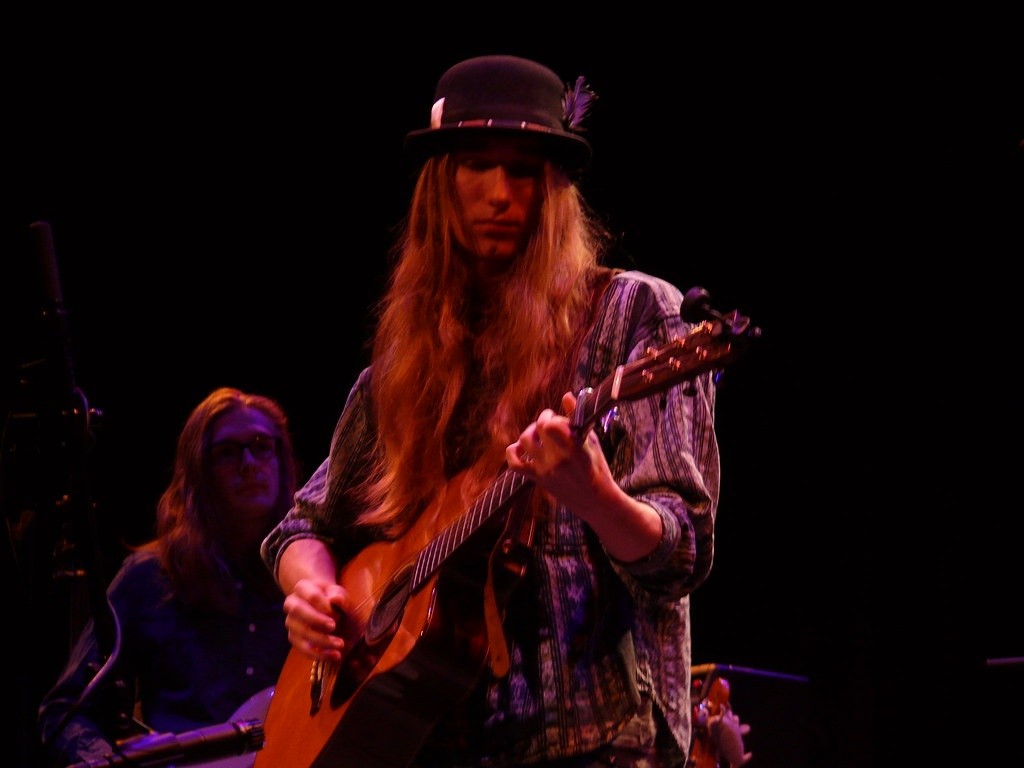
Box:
[207,433,279,467]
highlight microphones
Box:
[26,221,75,372]
[63,715,266,768]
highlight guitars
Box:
[255,303,769,768]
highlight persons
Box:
[260,57,726,768]
[34,388,302,768]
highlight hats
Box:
[405,53,599,168]
